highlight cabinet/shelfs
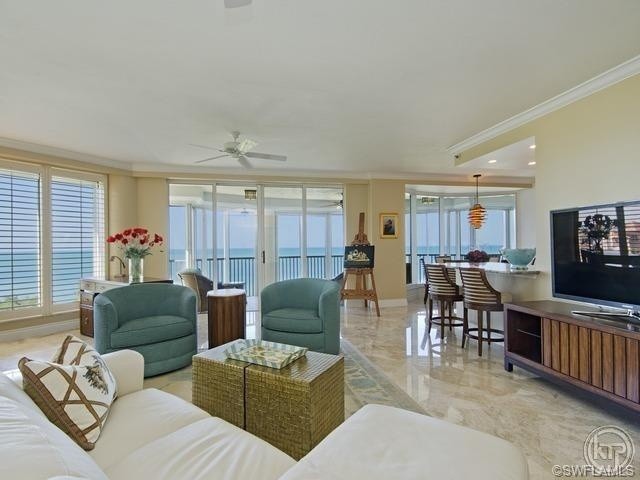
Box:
[502,299,639,423]
[76,277,174,340]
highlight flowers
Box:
[578,211,626,245]
[106,226,169,281]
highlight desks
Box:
[582,248,639,269]
[190,336,345,461]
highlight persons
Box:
[384,219,394,235]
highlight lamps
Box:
[422,196,435,206]
[468,173,488,230]
[244,189,258,201]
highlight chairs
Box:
[92,282,198,376]
[261,277,342,358]
[180,266,247,312]
[419,252,509,357]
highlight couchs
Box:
[0,349,530,478]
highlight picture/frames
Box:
[379,213,400,239]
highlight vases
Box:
[595,243,600,251]
[128,257,143,285]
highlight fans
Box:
[318,192,344,210]
[187,131,289,172]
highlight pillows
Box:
[16,333,117,448]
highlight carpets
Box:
[141,329,433,417]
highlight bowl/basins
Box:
[504,248,536,270]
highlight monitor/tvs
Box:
[549,199,640,323]
